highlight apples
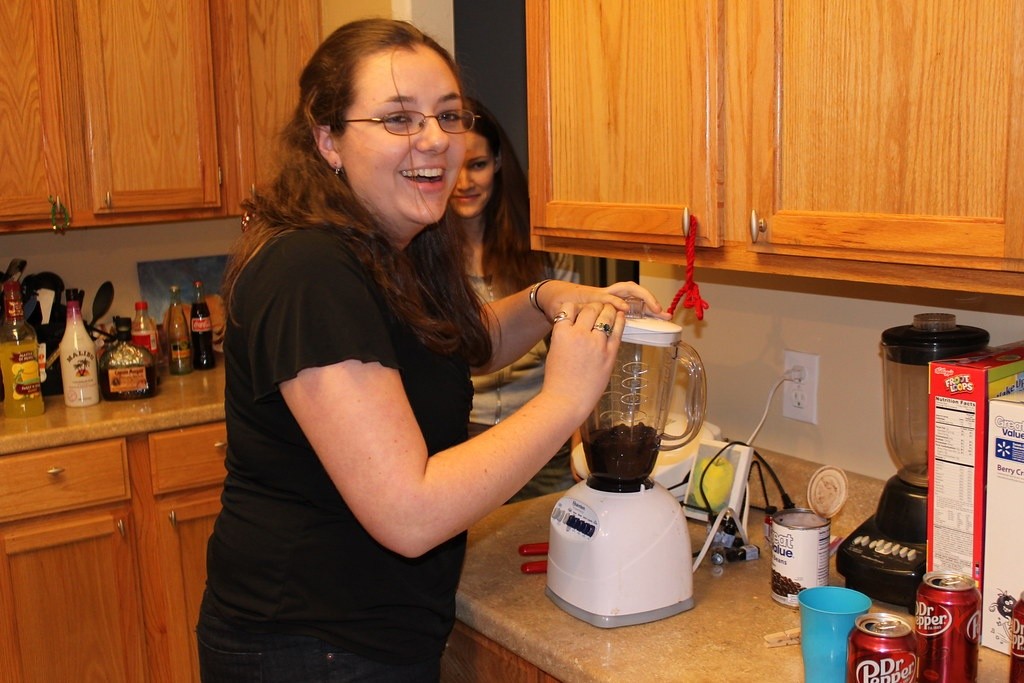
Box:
[692,454,733,509]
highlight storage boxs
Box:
[925,340,1023,584]
[980,388,1024,654]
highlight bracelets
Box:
[529,279,553,313]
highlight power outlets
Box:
[783,348,820,425]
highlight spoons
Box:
[88,281,114,332]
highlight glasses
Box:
[329,107,481,137]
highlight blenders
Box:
[542,297,706,629]
[836,313,990,617]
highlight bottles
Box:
[190,282,215,371]
[97,315,156,401]
[0,281,44,418]
[167,286,192,375]
[131,301,161,386]
[59,288,99,407]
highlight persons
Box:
[201,16,665,683]
[449,93,591,511]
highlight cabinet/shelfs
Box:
[0,0,391,239]
[0,420,243,682]
[522,0,1024,300]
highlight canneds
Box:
[770,465,847,607]
[846,612,918,683]
[915,570,982,683]
[1007,591,1024,683]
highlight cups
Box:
[798,586,872,683]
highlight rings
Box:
[592,322,612,336]
[551,309,575,322]
